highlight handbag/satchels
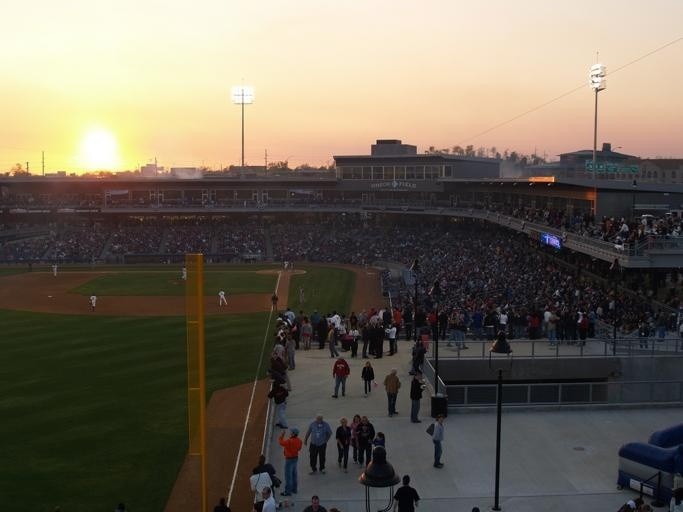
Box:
[426,423,433,436]
[271,473,281,488]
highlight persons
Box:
[1,192,366,266]
[182,267,186,280]
[362,192,682,338]
[90,294,97,311]
[272,292,278,311]
[299,285,305,303]
[251,307,683,512]
[52,264,57,278]
[219,290,227,306]
[284,259,294,272]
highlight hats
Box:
[290,427,299,435]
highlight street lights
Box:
[609,257,624,354]
[411,259,422,344]
[429,278,449,394]
[487,331,514,512]
[590,63,607,180]
